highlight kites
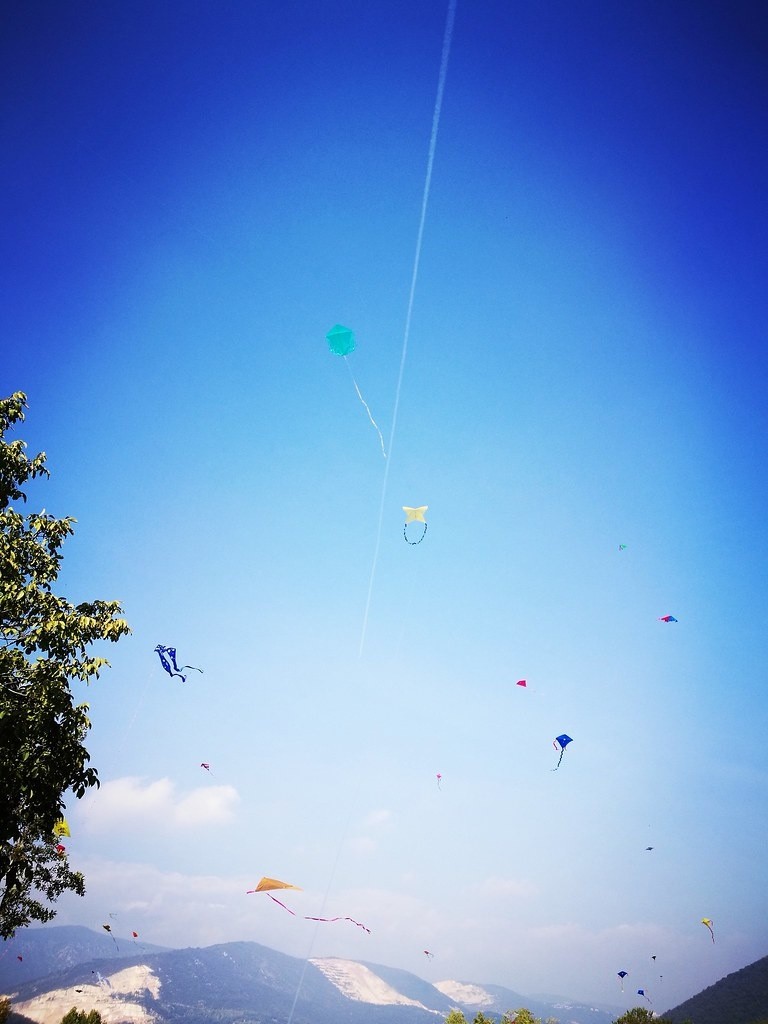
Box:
[16,326,716,1004]
[153,644,203,682]
